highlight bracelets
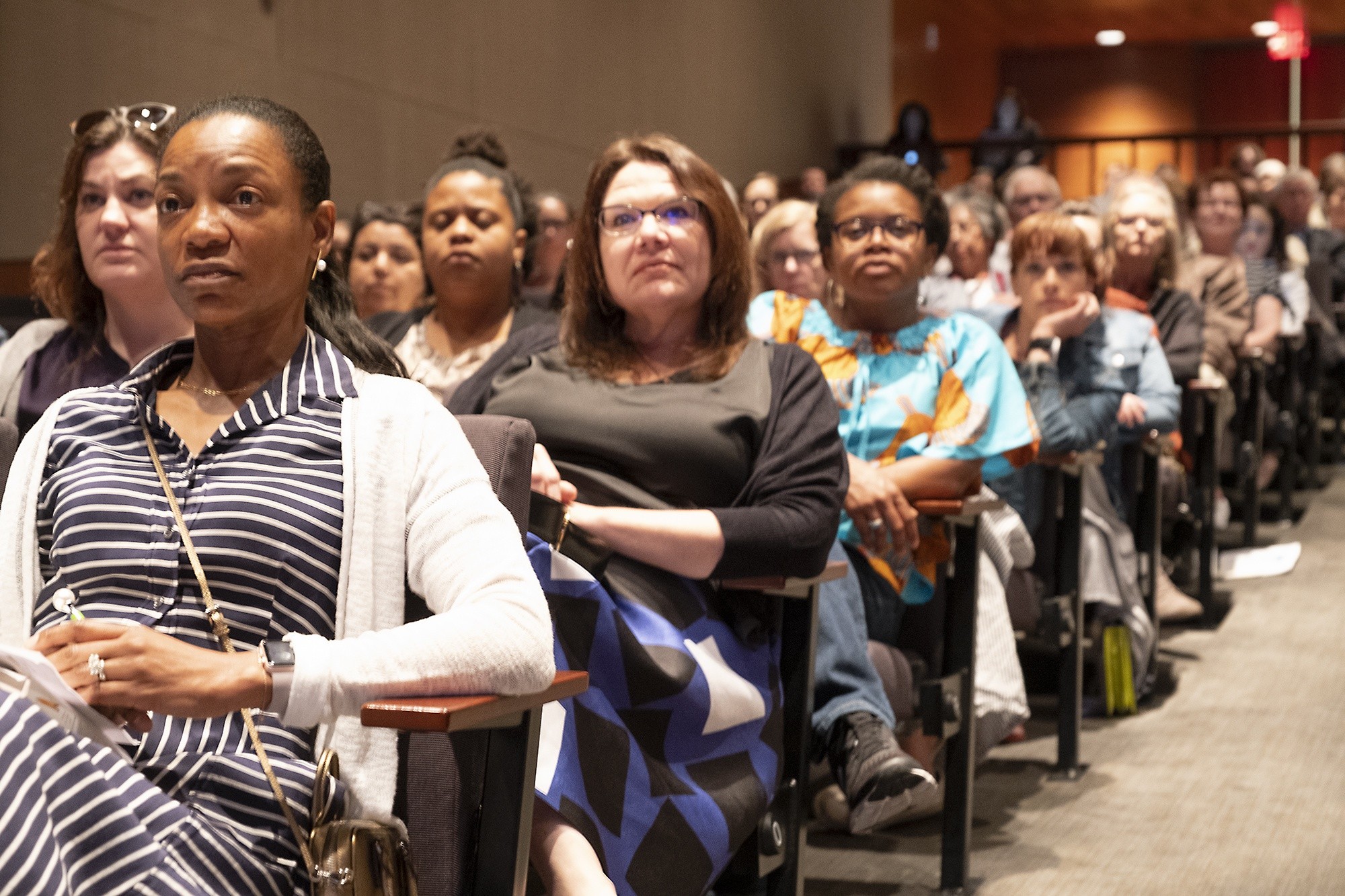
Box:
[1026,334,1061,361]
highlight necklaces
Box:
[179,369,261,396]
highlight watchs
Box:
[260,639,295,713]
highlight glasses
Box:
[596,198,700,234]
[70,101,177,138]
[754,246,819,272]
[831,217,923,247]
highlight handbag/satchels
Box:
[1102,624,1138,718]
[302,748,420,896]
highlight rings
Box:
[88,653,106,681]
[868,517,884,532]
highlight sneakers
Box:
[826,709,937,838]
[1154,567,1204,621]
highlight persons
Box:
[745,159,1043,833]
[743,144,1345,617]
[0,97,558,896]
[363,130,564,414]
[342,201,435,323]
[746,197,845,328]
[966,210,1181,745]
[481,134,851,896]
[329,216,355,276]
[0,102,176,503]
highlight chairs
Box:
[364,315,1344,895]
